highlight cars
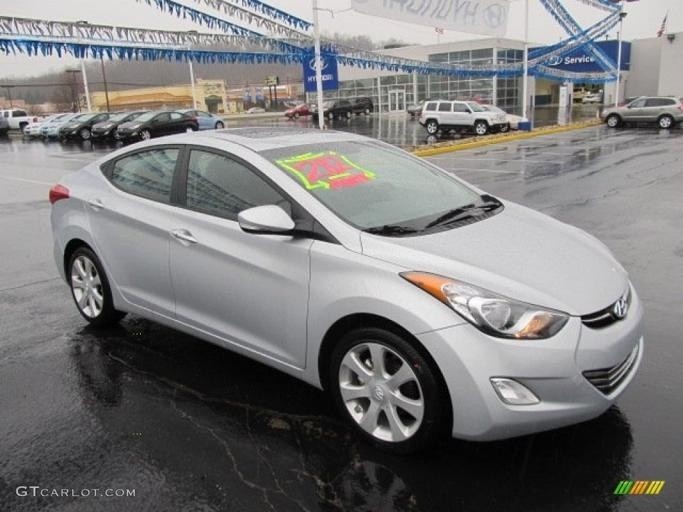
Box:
[408,97,431,118]
[50,126,644,444]
[39,113,77,142]
[90,111,148,141]
[602,96,683,107]
[283,103,315,121]
[583,88,603,103]
[117,111,199,143]
[22,115,53,139]
[0,113,8,136]
[68,322,632,512]
[173,109,224,129]
[58,112,116,140]
[482,104,528,133]
[245,107,265,114]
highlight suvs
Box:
[310,99,351,120]
[418,101,507,136]
[600,95,683,130]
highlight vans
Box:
[348,96,373,116]
[573,88,587,102]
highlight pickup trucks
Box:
[0,109,43,132]
